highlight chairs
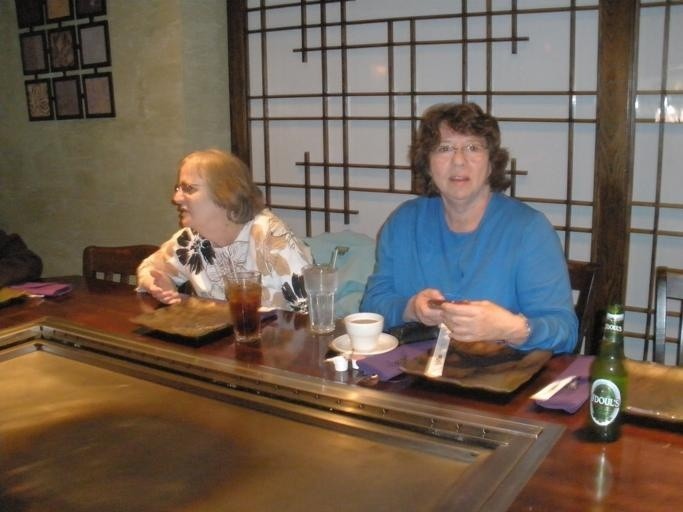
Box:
[566,260,601,356]
[82,245,192,295]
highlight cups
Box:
[224,271,262,342]
[301,264,338,333]
[344,312,384,351]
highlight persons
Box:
[135,149,319,314]
[359,103,578,353]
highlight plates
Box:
[329,332,399,355]
[128,296,276,339]
[400,340,554,396]
[0,286,25,302]
[621,360,683,428]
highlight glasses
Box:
[174,182,212,194]
[428,143,492,157]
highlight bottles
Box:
[588,303,629,443]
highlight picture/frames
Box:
[14,1,117,122]
